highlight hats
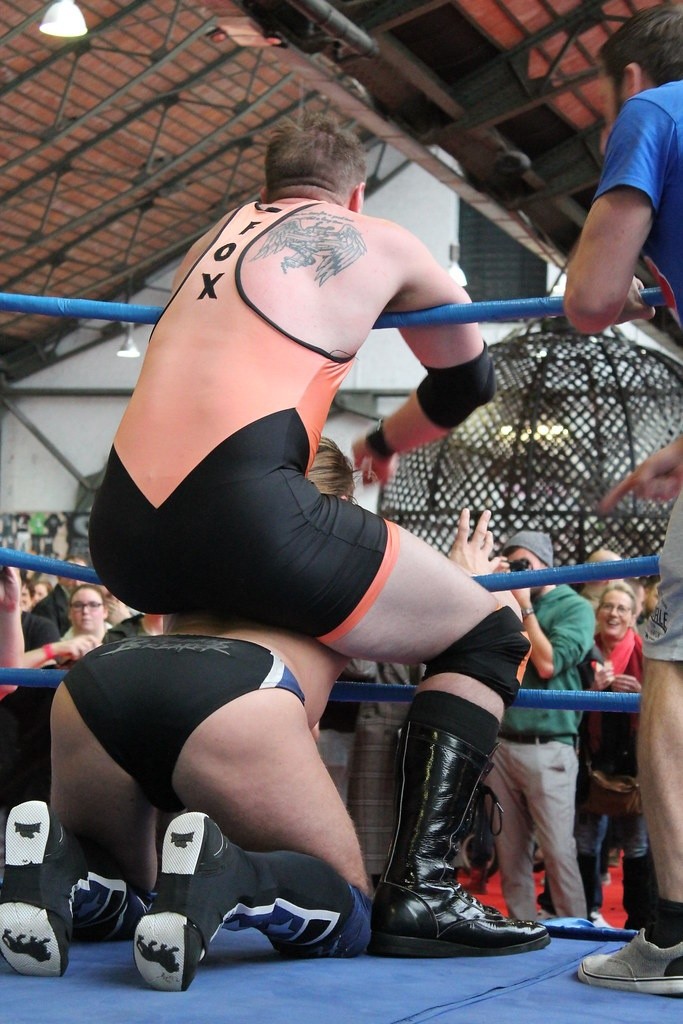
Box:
[501,530,554,568]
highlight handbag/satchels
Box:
[579,770,644,818]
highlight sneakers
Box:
[133,811,257,991]
[0,800,89,977]
[578,928,683,994]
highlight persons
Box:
[87,115,552,958]
[563,5,683,995]
[2,529,661,939]
[1,435,509,991]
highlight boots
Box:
[367,722,552,956]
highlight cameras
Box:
[508,558,533,573]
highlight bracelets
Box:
[521,608,533,616]
[42,642,54,659]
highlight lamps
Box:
[38,0,89,38]
[116,322,140,358]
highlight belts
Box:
[497,731,550,744]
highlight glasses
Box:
[602,601,632,616]
[71,600,104,612]
